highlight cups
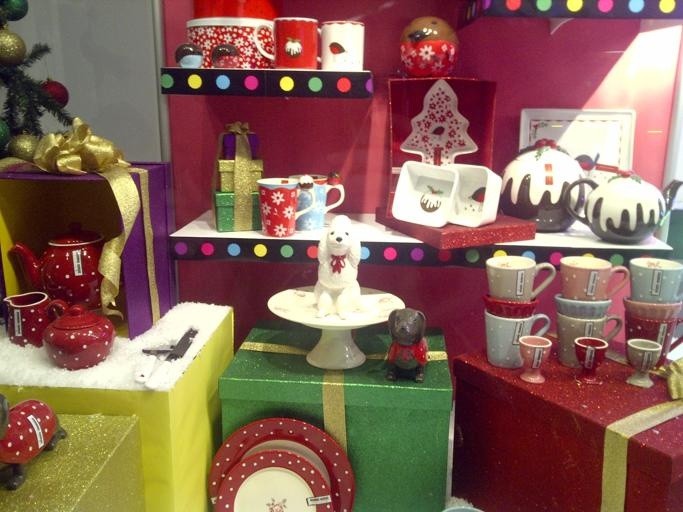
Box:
[317,20,365,70]
[554,255,631,368]
[256,175,315,237]
[289,171,345,232]
[2,291,70,348]
[623,257,683,367]
[482,254,556,369]
[253,17,318,69]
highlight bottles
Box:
[43,298,115,370]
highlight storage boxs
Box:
[218,321,453,512]
[1,161,177,340]
[451,332,683,511]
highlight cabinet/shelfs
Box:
[151,0,683,355]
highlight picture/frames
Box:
[519,108,636,171]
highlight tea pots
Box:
[7,220,124,310]
[562,171,683,244]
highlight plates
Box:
[207,417,355,512]
[216,450,334,512]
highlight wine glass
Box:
[573,336,609,385]
[518,335,553,384]
[626,338,662,388]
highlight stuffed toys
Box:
[313,215,363,320]
